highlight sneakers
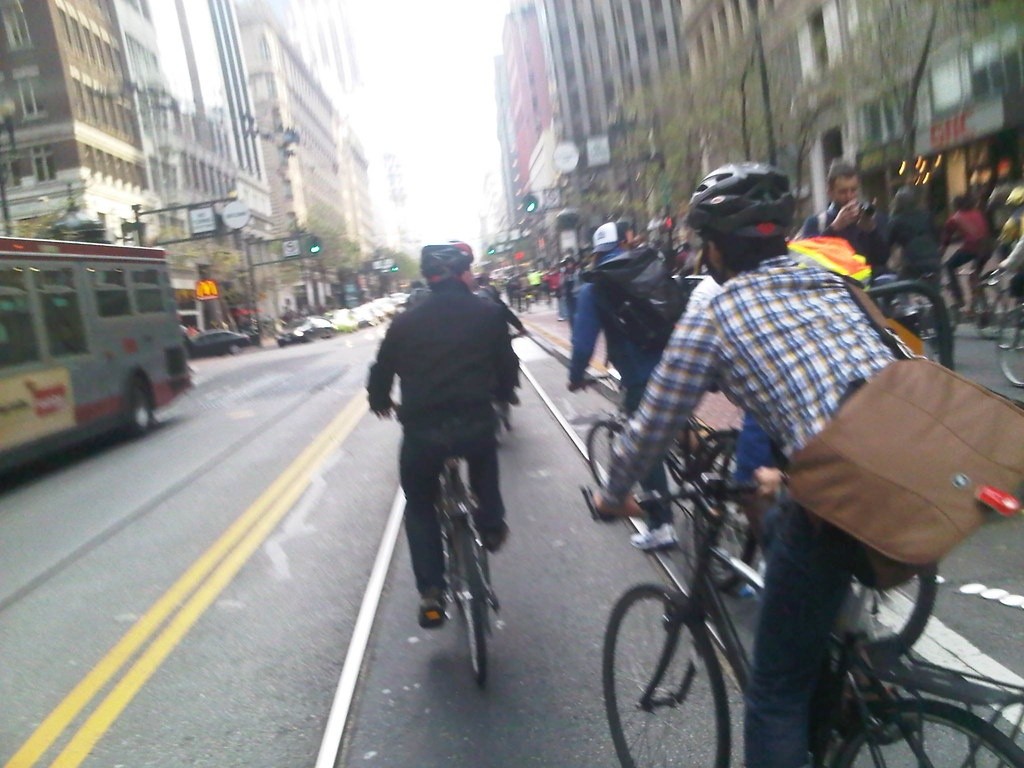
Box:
[629,522,678,550]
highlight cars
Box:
[187,329,251,358]
[275,292,410,347]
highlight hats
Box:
[589,221,630,256]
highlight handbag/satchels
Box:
[787,360,1024,567]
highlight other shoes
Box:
[418,586,446,629]
[483,521,508,552]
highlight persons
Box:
[408,280,428,308]
[186,294,373,335]
[802,165,1024,326]
[367,241,521,627]
[472,219,709,549]
[595,162,898,768]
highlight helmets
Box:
[1005,186,1024,205]
[683,162,797,237]
[420,241,474,285]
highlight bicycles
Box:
[389,399,513,687]
[930,266,1024,387]
[568,373,1024,768]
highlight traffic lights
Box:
[310,244,320,254]
[489,250,495,255]
[524,201,538,212]
[392,266,399,271]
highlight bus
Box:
[1,236,194,485]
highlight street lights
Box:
[0,99,17,235]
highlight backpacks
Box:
[581,244,691,352]
